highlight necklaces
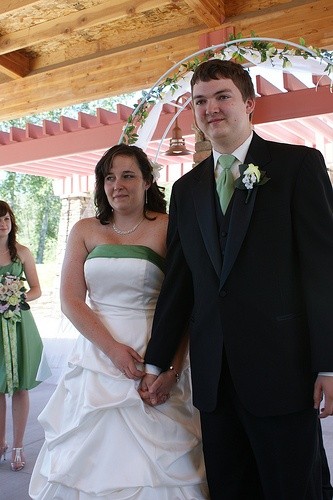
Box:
[111,210,150,235]
[0,249,10,256]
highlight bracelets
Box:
[169,364,180,383]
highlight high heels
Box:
[0,443,8,460]
[11,448,25,471]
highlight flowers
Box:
[233,162,271,204]
[0,272,31,325]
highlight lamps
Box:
[160,96,205,156]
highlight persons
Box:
[29,144,213,500]
[0,200,52,470]
[137,58,333,500]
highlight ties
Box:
[216,154,236,216]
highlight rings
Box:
[123,372,126,375]
